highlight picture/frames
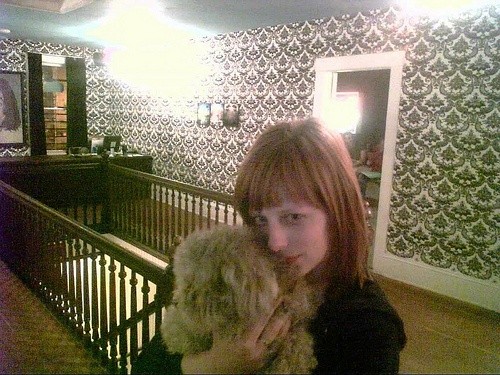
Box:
[0,71,27,148]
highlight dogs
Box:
[159,224,328,375]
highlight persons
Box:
[130,119,407,375]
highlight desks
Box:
[0,152,153,203]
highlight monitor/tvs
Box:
[102,135,121,153]
